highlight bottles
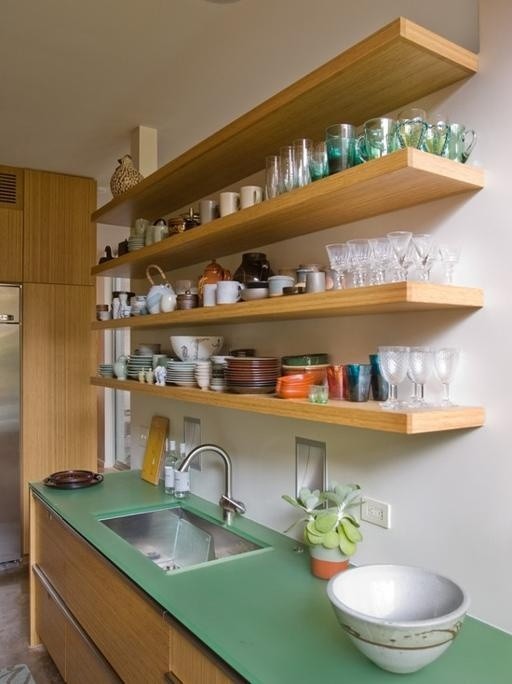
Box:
[165,441,177,495]
[173,443,191,498]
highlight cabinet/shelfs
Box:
[21,284,104,554]
[31,472,512,684]
[0,161,24,283]
[23,162,99,286]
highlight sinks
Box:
[98,505,272,573]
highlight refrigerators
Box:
[0,283,23,565]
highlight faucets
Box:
[178,442,247,521]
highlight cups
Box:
[325,123,357,174]
[356,118,398,165]
[292,138,312,187]
[216,281,245,305]
[326,364,349,401]
[369,354,390,401]
[306,139,330,183]
[405,346,431,409]
[157,356,178,368]
[421,112,448,156]
[347,364,373,403]
[265,155,279,200]
[347,239,370,288]
[152,354,168,370]
[433,347,458,407]
[240,280,269,301]
[305,270,326,294]
[442,121,477,165]
[279,145,293,196]
[325,243,351,290]
[219,192,240,218]
[367,236,394,286]
[199,200,219,226]
[202,283,217,307]
[240,186,263,209]
[412,234,435,284]
[378,345,407,410]
[387,230,413,283]
[438,244,461,285]
[395,107,428,150]
[160,294,179,314]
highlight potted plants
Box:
[276,479,366,583]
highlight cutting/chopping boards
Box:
[141,416,169,487]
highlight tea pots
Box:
[197,259,234,307]
[145,264,178,314]
[234,252,275,284]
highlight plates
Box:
[275,374,324,400]
[126,354,153,379]
[210,356,224,391]
[195,361,210,389]
[43,474,105,489]
[100,364,114,376]
[165,361,195,387]
[49,470,93,485]
[223,357,280,394]
[129,294,150,317]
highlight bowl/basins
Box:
[127,218,150,252]
[327,563,471,674]
[146,226,169,246]
[95,304,109,321]
[138,344,160,356]
[170,335,224,361]
